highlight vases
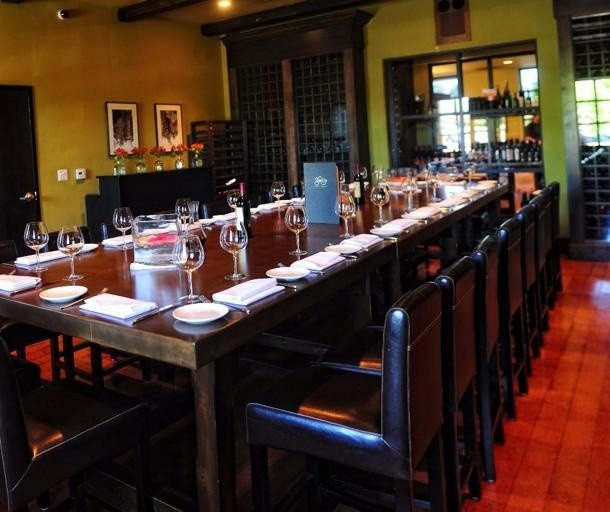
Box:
[114,154,203,176]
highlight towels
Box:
[80,293,159,327]
[14,251,66,268]
[0,274,40,295]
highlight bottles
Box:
[467,137,542,163]
[494,79,539,109]
[236,182,250,240]
[413,146,463,165]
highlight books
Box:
[303,162,341,225]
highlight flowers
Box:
[114,143,204,163]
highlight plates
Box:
[38,285,88,303]
[172,304,230,322]
[265,266,310,282]
[340,162,498,240]
[192,182,305,239]
[79,243,99,254]
[321,244,361,255]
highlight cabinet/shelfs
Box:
[190,119,250,203]
[401,104,543,179]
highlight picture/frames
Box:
[154,103,184,154]
[105,101,141,157]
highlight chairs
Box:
[0,338,157,512]
[42,226,152,389]
[246,181,562,510]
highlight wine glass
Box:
[56,224,85,281]
[113,207,135,250]
[172,235,205,303]
[272,180,286,211]
[227,188,242,224]
[174,198,192,236]
[336,192,356,238]
[400,178,418,212]
[219,223,249,280]
[286,205,309,256]
[24,220,51,274]
[370,185,390,223]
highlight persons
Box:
[524,116,542,143]
